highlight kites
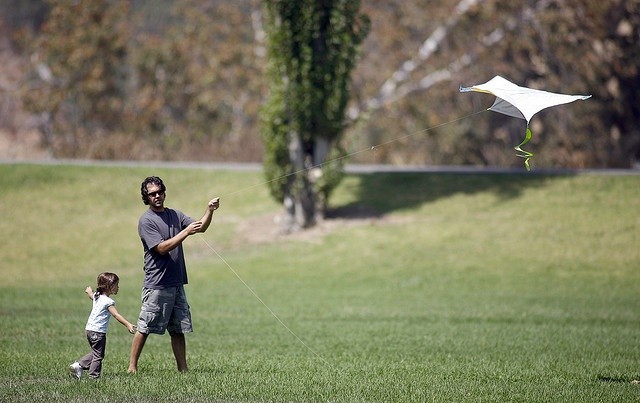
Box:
[459,73,593,174]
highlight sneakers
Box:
[69,362,83,385]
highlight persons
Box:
[125,175,220,374]
[68,272,138,382]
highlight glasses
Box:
[147,189,164,197]
